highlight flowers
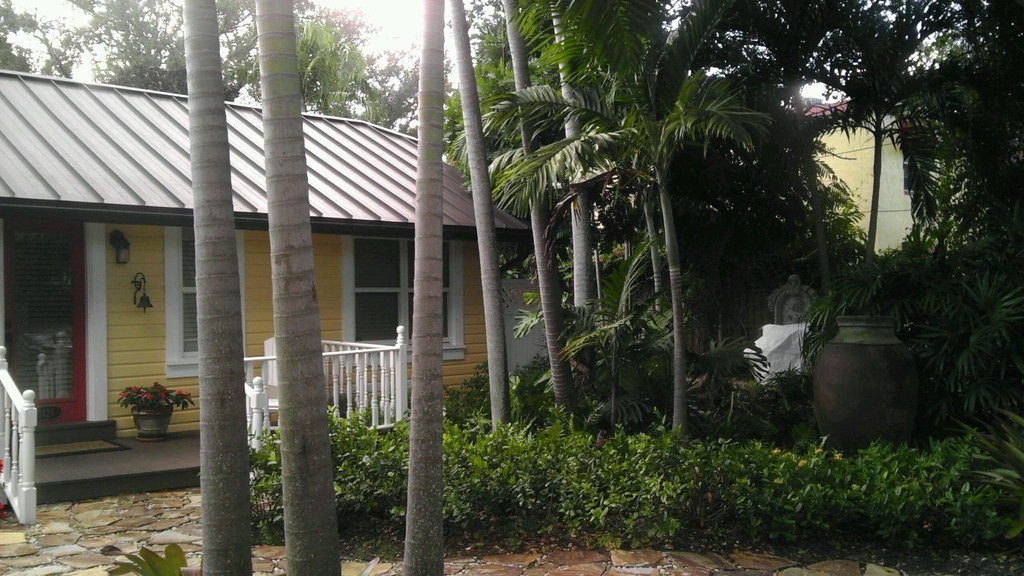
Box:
[117,381,195,414]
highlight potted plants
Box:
[129,400,176,445]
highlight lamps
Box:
[132,271,155,313]
[107,228,130,264]
